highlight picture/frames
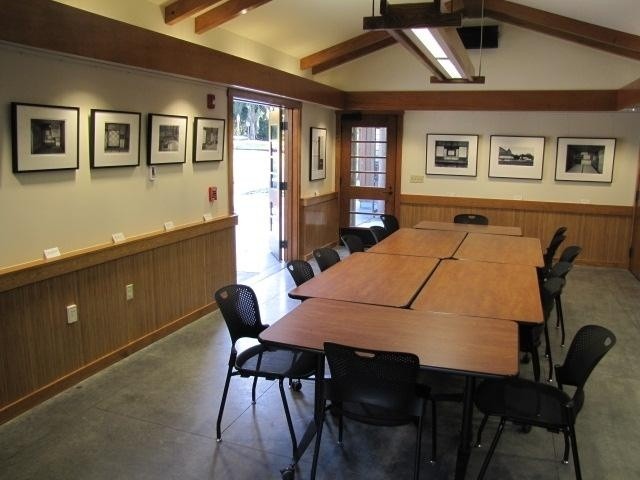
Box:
[148,112,188,165]
[488,135,546,180]
[554,137,617,183]
[91,109,141,168]
[426,134,478,177]
[193,117,226,163]
[310,127,327,181]
[12,102,79,174]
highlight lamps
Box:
[361,0,490,85]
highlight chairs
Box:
[452,212,490,225]
[519,223,581,385]
[210,283,316,460]
[473,321,618,479]
[285,211,405,303]
[311,335,442,479]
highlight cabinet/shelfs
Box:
[257,295,519,476]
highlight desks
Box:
[453,232,545,269]
[411,258,545,324]
[415,219,523,237]
[364,226,469,259]
[288,252,438,310]
